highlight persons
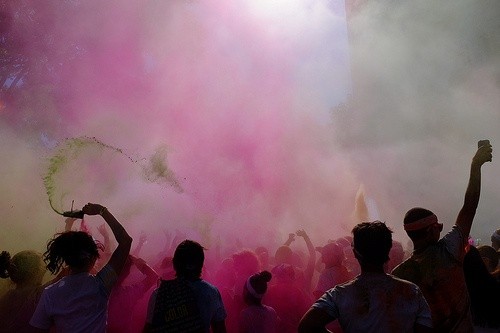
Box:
[137,238,228,333]
[386,238,404,276]
[461,226,500,333]
[55,207,363,307]
[0,214,75,333]
[138,238,229,332]
[298,220,433,332]
[231,270,285,333]
[387,146,493,333]
[28,201,134,333]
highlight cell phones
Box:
[479,140,491,162]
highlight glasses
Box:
[427,223,443,232]
